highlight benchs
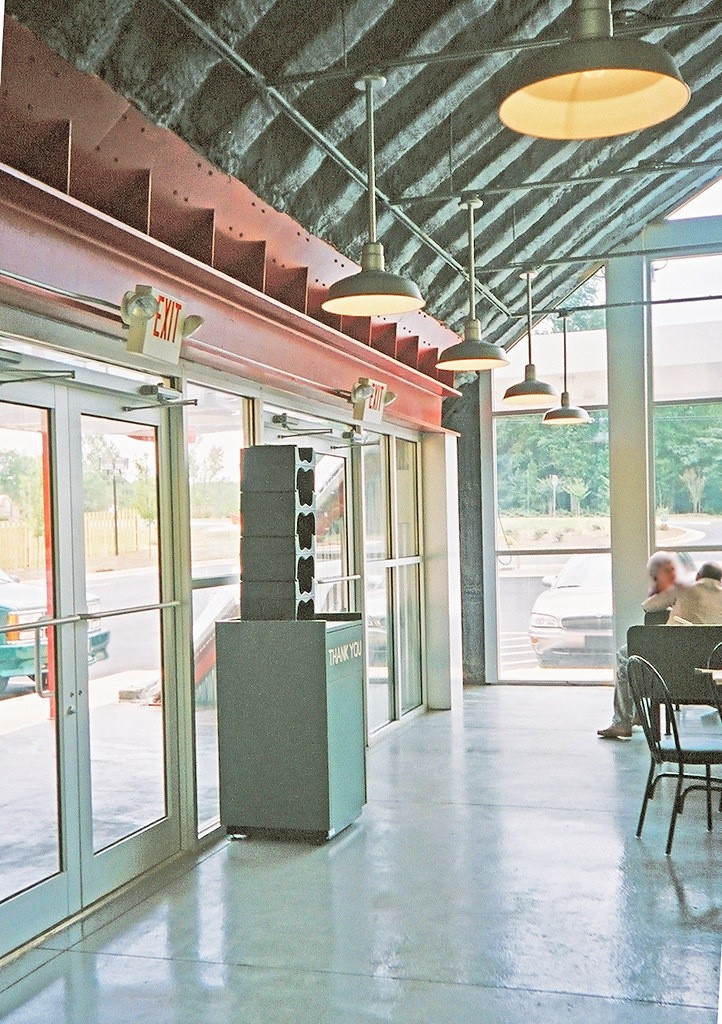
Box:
[628,625,722,712]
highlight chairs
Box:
[706,643,722,812]
[625,654,721,856]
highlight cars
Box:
[366,578,389,654]
[529,546,698,667]
[0,569,110,694]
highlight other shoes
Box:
[597,723,633,737]
[630,714,643,726]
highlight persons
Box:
[642,552,688,600]
[596,561,722,737]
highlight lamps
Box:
[122,286,204,366]
[504,267,557,407]
[542,312,589,425]
[351,378,397,423]
[436,196,509,372]
[321,69,426,317]
[497,0,691,140]
